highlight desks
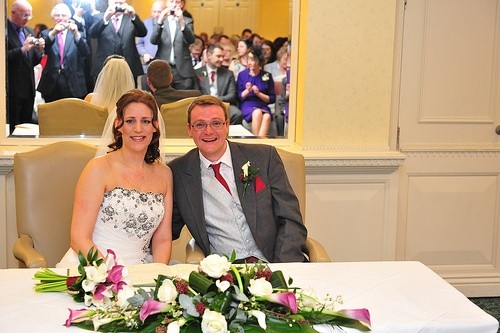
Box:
[11,123,253,136]
[1,259,500,333]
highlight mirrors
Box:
[0,0,300,147]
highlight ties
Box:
[58,30,64,65]
[208,161,232,196]
[211,72,216,82]
[113,16,120,33]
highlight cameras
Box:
[169,9,177,15]
[62,23,69,29]
[28,37,40,47]
[115,6,125,12]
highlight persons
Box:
[34,0,291,136]
[87,0,148,89]
[83,54,136,115]
[149,95,310,264]
[195,43,244,125]
[52,88,183,268]
[6,0,46,135]
[150,0,196,90]
[36,2,91,103]
[146,58,201,110]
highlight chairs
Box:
[13,140,111,269]
[185,147,332,264]
[241,104,277,136]
[159,98,230,139]
[35,98,108,135]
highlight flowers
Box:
[34,248,127,307]
[261,72,269,82]
[64,250,372,333]
[197,71,207,82]
[238,160,261,202]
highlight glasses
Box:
[188,120,226,131]
[19,14,33,20]
